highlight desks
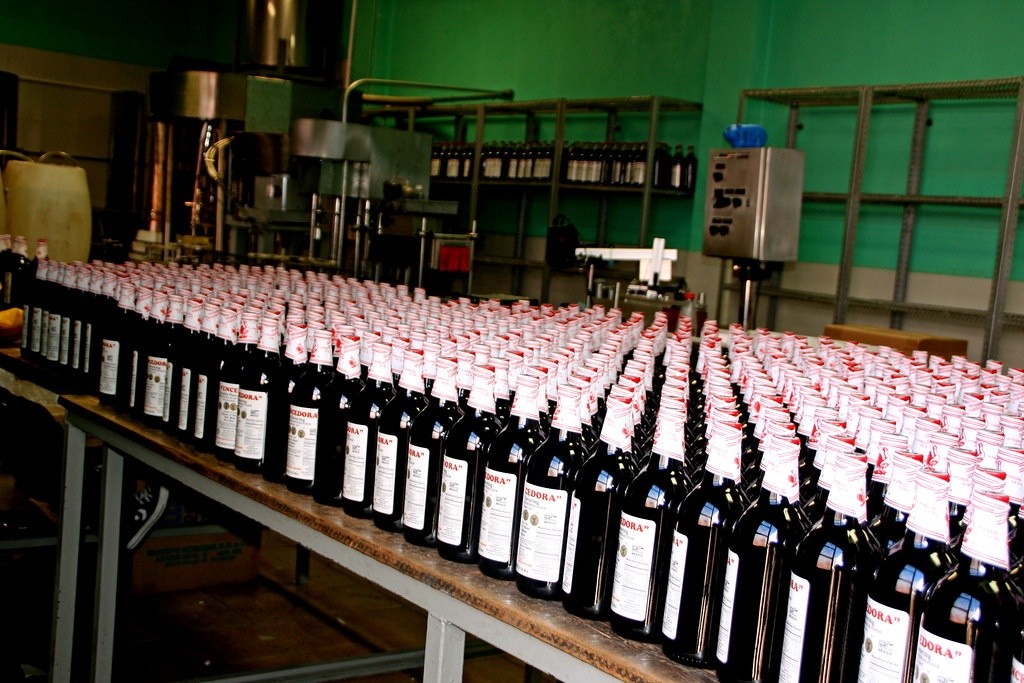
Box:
[0,338,102,683]
[42,395,721,683]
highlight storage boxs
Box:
[823,323,968,359]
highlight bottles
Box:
[0,231,1023,683]
[432,142,698,189]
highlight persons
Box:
[0,306,169,550]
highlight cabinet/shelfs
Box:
[360,94,704,317]
[712,76,1024,368]
